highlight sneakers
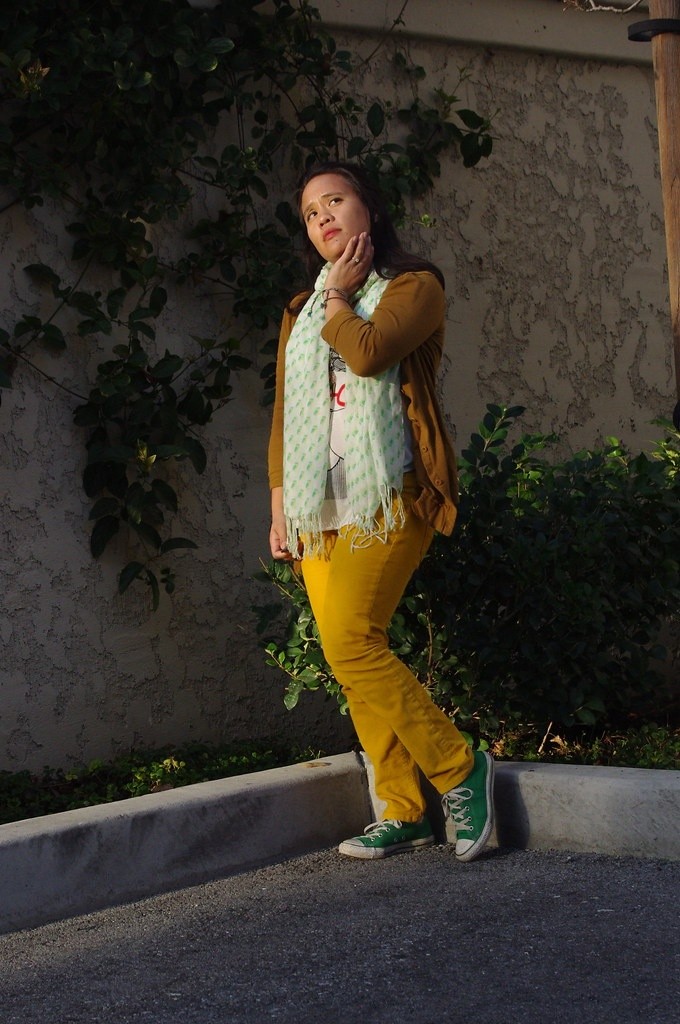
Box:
[338,814,433,859]
[440,750,495,863]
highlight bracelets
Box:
[308,287,350,317]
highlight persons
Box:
[268,162,496,863]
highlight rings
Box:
[352,255,360,263]
